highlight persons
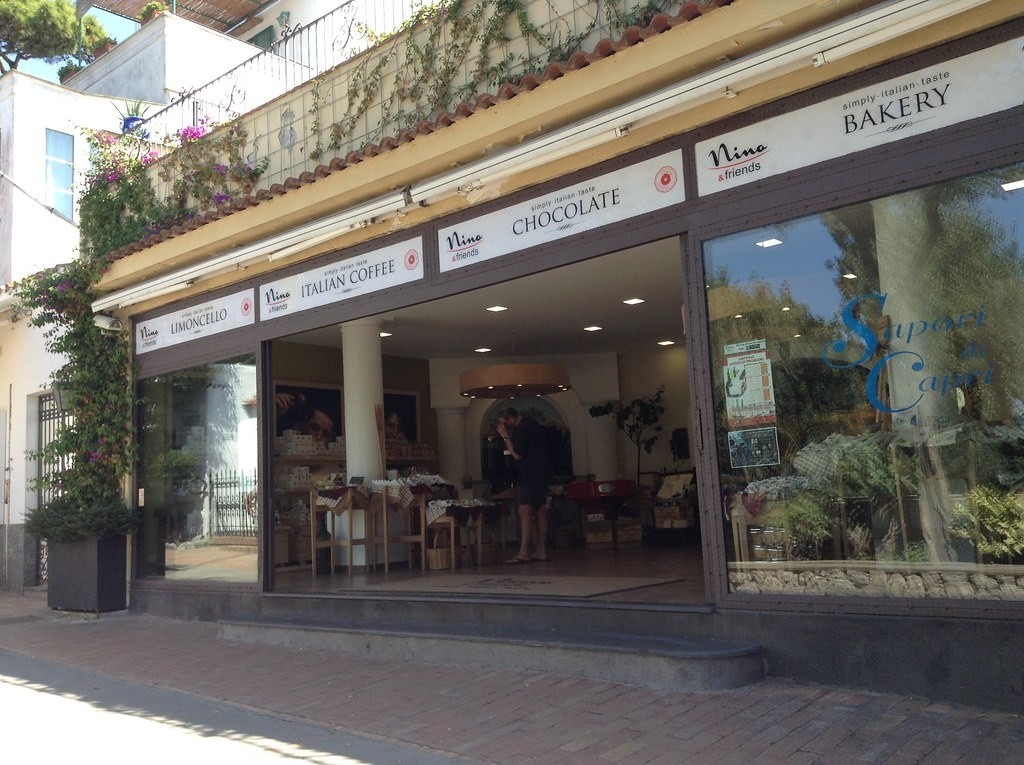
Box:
[275,391,334,444]
[495,408,548,565]
[384,409,407,439]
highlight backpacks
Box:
[531,424,573,497]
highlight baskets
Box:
[467,525,497,564]
[426,529,459,570]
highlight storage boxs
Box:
[581,512,643,544]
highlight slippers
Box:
[505,557,534,566]
[529,552,548,562]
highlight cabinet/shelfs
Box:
[271,456,507,577]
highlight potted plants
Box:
[59,64,84,85]
[616,385,667,510]
[91,36,117,60]
[135,1,168,28]
[111,98,151,140]
[27,494,144,612]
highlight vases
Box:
[749,526,790,561]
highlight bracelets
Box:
[503,435,511,440]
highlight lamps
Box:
[459,327,572,401]
